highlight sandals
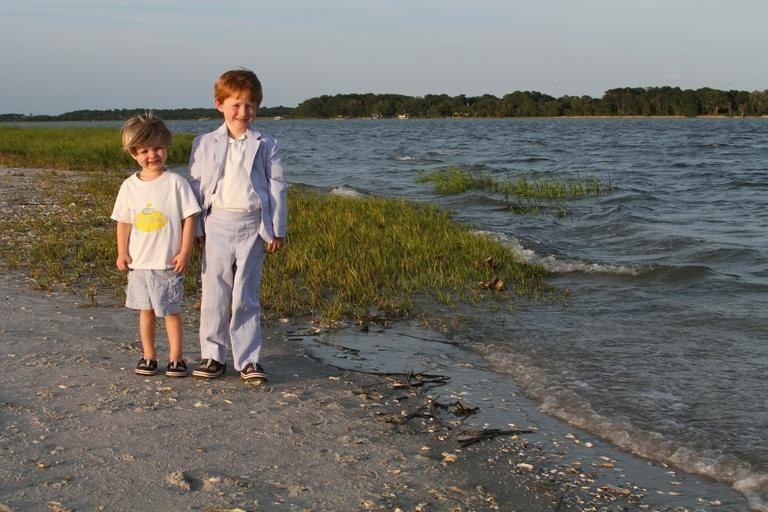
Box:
[239,362,269,380]
[165,360,188,376]
[191,358,227,379]
[135,357,158,376]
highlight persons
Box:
[111,112,203,377]
[187,70,289,381]
